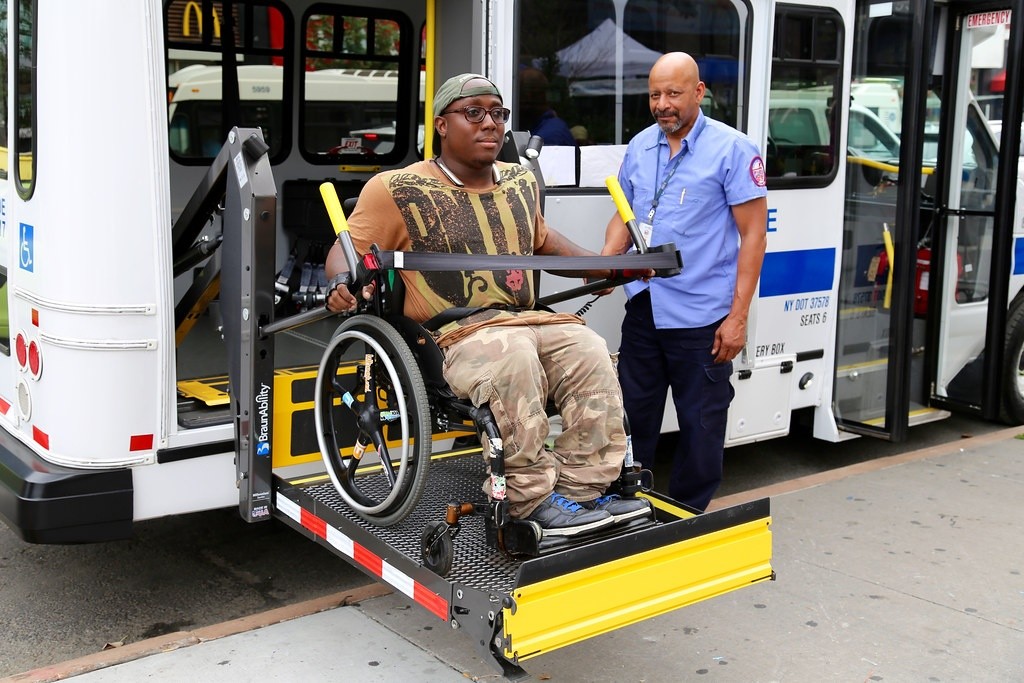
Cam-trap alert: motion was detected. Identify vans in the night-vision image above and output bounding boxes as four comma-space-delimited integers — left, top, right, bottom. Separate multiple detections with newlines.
782, 83, 900, 146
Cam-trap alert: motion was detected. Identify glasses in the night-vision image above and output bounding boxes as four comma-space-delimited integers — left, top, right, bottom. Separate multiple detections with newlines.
440, 105, 510, 125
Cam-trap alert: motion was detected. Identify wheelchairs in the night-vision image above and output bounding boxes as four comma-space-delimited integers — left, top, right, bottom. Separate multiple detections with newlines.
314, 181, 683, 576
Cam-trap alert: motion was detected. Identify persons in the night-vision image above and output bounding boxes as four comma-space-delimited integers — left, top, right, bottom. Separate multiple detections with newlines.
324, 73, 656, 536
584, 52, 767, 512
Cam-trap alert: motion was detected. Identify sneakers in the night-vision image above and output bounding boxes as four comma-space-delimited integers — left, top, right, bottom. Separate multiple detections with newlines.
576, 495, 653, 526
525, 494, 615, 536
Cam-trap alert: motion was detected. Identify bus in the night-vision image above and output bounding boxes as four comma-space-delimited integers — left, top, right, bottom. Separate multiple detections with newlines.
167, 64, 426, 158
0, 0, 1023, 545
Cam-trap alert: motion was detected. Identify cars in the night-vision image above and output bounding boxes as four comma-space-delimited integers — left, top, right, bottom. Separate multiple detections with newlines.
976, 95, 1024, 156
769, 99, 900, 163
327, 121, 426, 157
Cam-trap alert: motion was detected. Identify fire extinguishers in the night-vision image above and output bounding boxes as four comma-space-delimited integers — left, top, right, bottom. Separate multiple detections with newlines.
911, 237, 963, 319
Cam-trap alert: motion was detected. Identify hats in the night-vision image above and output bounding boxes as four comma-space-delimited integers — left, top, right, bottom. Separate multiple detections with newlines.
432, 73, 504, 120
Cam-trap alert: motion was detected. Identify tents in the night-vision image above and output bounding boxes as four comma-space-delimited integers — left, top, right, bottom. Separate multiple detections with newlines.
531, 14, 666, 87
